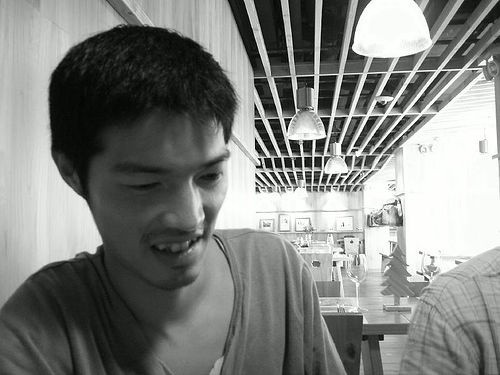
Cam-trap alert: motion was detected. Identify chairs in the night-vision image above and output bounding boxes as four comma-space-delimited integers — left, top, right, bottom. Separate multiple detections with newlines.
302, 252, 363, 375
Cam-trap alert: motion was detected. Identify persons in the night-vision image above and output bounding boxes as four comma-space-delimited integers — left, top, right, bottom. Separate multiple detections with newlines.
1, 23, 348, 375
399, 245, 500, 375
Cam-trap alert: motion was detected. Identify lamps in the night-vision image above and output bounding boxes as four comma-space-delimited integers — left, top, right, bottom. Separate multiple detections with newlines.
324, 141, 349, 175
287, 85, 326, 141
483, 61, 500, 83
351, 0, 432, 57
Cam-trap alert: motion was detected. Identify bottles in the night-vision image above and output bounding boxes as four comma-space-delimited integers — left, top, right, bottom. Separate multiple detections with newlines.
327, 234, 333, 247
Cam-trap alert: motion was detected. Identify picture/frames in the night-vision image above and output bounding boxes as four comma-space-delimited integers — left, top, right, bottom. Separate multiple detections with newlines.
278, 213, 290, 232
294, 217, 311, 231
259, 219, 274, 232
335, 216, 354, 232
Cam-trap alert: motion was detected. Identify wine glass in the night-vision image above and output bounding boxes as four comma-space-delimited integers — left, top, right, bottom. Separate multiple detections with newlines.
345, 254, 368, 313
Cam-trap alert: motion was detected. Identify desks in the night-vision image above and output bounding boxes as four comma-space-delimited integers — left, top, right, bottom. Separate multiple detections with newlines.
313, 252, 349, 297
319, 297, 417, 375
